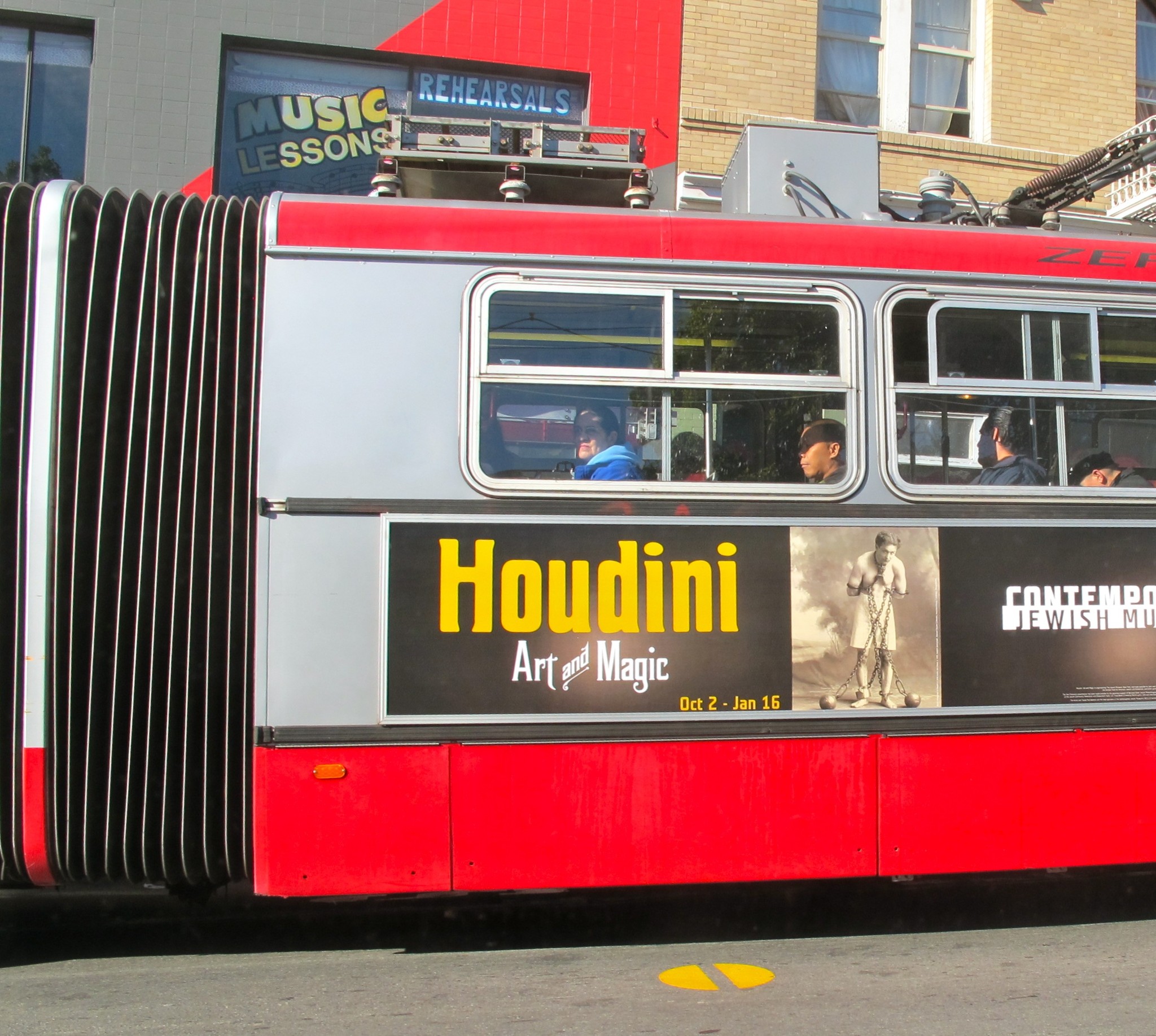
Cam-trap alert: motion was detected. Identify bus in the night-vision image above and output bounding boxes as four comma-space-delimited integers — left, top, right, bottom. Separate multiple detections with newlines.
3, 171, 1156, 904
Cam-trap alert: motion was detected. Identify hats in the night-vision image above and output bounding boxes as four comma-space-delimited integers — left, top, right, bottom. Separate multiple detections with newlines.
1069, 446, 1114, 487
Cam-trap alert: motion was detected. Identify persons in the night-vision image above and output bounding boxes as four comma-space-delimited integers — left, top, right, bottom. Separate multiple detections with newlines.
479, 415, 508, 478
570, 404, 646, 481
967, 406, 1048, 486
796, 418, 847, 485
671, 432, 706, 482
1067, 447, 1154, 488
846, 531, 909, 709
